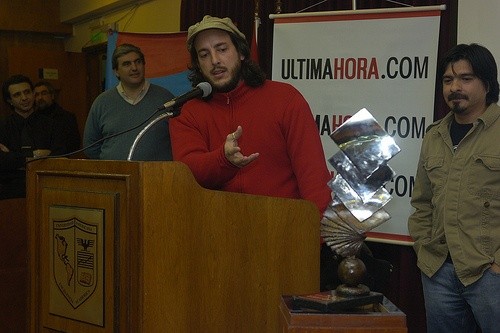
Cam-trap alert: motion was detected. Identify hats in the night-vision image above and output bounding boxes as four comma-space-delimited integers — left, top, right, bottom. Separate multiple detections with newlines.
187, 15, 246, 56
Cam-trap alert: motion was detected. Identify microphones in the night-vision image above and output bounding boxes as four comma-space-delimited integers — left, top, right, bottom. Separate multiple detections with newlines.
158, 82, 212, 111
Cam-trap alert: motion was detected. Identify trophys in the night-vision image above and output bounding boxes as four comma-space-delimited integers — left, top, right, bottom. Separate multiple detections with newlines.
295, 107, 401, 311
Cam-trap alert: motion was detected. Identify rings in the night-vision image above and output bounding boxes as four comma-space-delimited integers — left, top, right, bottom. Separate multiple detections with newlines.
232, 132, 237, 140
408, 41, 500, 333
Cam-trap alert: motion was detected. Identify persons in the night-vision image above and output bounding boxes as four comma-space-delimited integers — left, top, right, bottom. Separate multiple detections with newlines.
0, 77, 72, 198
29, 80, 82, 157
168, 15, 334, 249
83, 42, 176, 161
